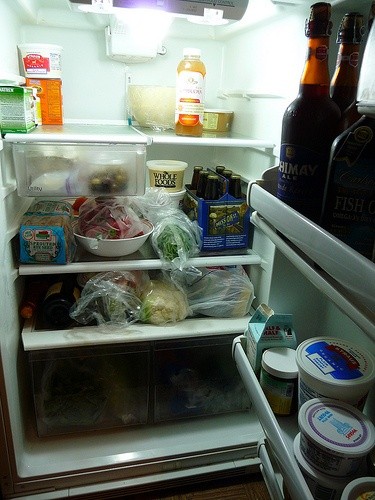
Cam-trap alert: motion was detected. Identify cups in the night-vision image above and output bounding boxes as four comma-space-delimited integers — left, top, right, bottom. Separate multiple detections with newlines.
293, 335, 375, 422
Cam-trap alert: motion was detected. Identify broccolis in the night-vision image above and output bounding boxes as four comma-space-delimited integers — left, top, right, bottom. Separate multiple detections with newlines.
137, 280, 189, 324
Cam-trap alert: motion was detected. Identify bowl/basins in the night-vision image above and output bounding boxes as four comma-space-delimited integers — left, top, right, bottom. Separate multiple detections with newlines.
74, 215, 154, 257
128, 85, 175, 129
291, 398, 375, 500
146, 158, 188, 212
18, 42, 65, 80
203, 108, 233, 133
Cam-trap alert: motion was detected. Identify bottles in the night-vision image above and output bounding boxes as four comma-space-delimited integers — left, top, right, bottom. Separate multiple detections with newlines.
19, 276, 80, 331
175, 46, 206, 138
277, 2, 346, 218
322, 112, 375, 260
327, 9, 366, 134
186, 164, 244, 250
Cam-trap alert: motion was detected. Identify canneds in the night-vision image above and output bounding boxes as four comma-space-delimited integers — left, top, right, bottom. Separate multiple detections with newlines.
173, 48, 234, 137
